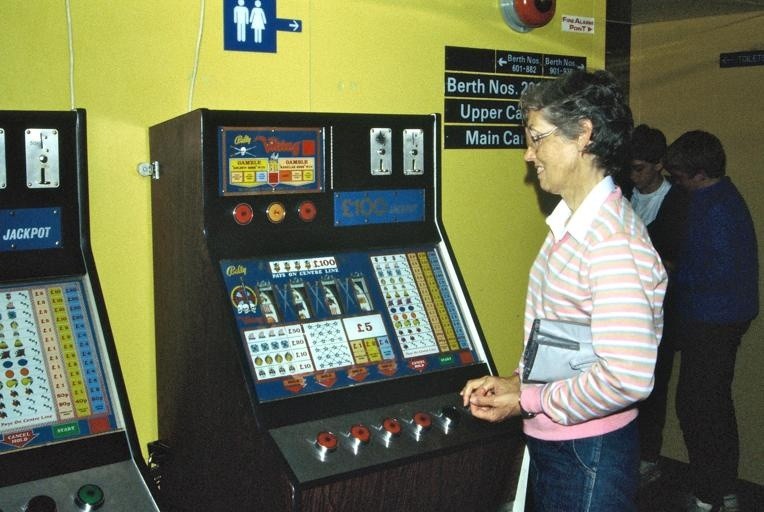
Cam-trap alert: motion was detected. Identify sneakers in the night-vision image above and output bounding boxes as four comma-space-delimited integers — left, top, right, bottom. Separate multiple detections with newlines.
685, 492, 740, 512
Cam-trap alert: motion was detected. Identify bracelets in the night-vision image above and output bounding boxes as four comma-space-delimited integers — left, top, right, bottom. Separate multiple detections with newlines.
517, 392, 537, 420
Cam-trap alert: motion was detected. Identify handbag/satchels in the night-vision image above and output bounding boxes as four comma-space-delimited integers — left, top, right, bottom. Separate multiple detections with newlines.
520, 316, 602, 385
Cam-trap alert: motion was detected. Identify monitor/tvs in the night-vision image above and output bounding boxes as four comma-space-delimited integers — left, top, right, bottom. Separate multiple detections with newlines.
0, 275, 120, 458
219, 243, 483, 404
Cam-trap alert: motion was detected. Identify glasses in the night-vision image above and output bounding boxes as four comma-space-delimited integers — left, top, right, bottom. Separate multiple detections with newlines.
523, 121, 560, 147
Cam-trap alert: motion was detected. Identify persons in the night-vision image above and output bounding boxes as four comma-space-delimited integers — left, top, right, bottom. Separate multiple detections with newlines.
456, 67, 674, 511
616, 122, 688, 492
660, 128, 760, 510
